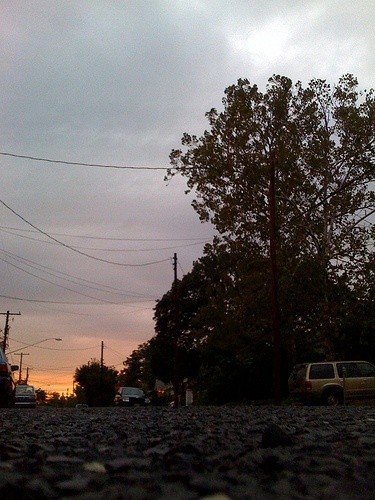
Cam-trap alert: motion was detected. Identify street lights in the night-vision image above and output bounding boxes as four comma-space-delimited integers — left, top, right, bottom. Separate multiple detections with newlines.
6, 337, 62, 355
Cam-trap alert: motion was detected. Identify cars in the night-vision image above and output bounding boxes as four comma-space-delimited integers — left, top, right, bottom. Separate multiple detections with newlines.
114, 387, 151, 407
13, 385, 37, 409
75, 404, 88, 408
288, 360, 375, 406
